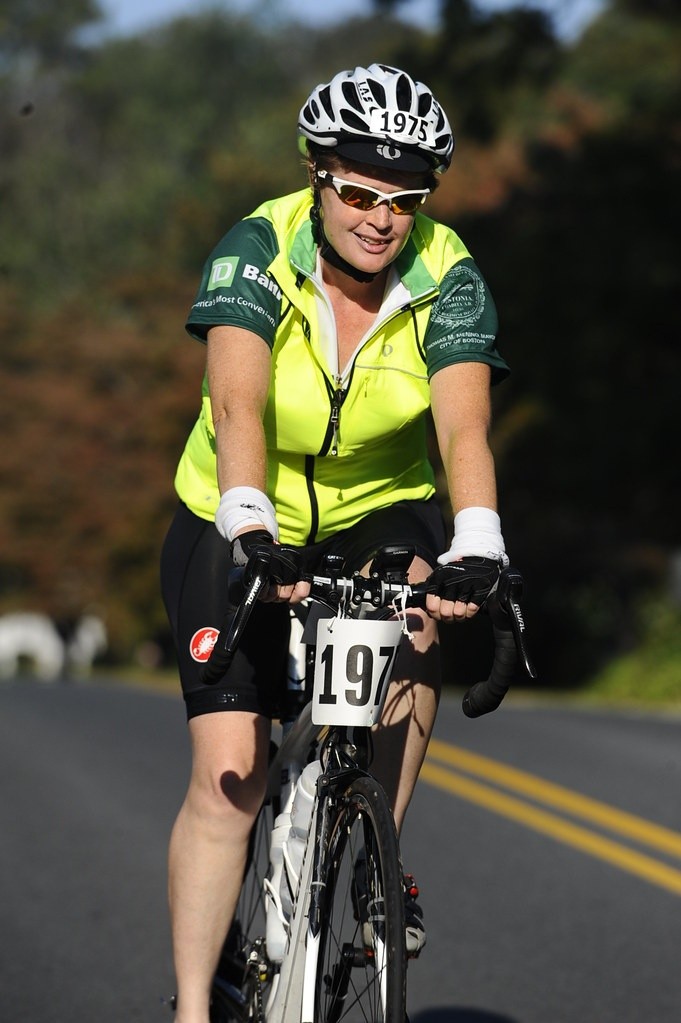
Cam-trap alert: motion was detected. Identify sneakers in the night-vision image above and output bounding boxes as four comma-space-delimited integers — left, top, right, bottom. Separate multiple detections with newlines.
351, 847, 425, 959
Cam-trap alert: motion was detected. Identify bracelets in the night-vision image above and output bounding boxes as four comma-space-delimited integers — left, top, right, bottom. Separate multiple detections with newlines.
454, 507, 501, 536
219, 486, 276, 536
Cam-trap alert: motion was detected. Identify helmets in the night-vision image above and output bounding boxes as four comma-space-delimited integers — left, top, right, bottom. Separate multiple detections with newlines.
296, 62, 453, 175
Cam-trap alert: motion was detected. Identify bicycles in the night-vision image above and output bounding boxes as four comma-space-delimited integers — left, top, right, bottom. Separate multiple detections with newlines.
171, 545, 534, 1023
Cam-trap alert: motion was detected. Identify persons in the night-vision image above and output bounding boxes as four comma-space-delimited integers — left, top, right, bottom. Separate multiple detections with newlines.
161, 62, 509, 1023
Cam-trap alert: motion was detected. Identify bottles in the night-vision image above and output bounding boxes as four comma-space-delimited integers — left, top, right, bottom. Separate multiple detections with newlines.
265, 791, 288, 965
278, 760, 325, 914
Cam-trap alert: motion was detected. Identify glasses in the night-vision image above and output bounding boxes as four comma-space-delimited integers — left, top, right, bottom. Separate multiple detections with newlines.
312, 167, 440, 215
